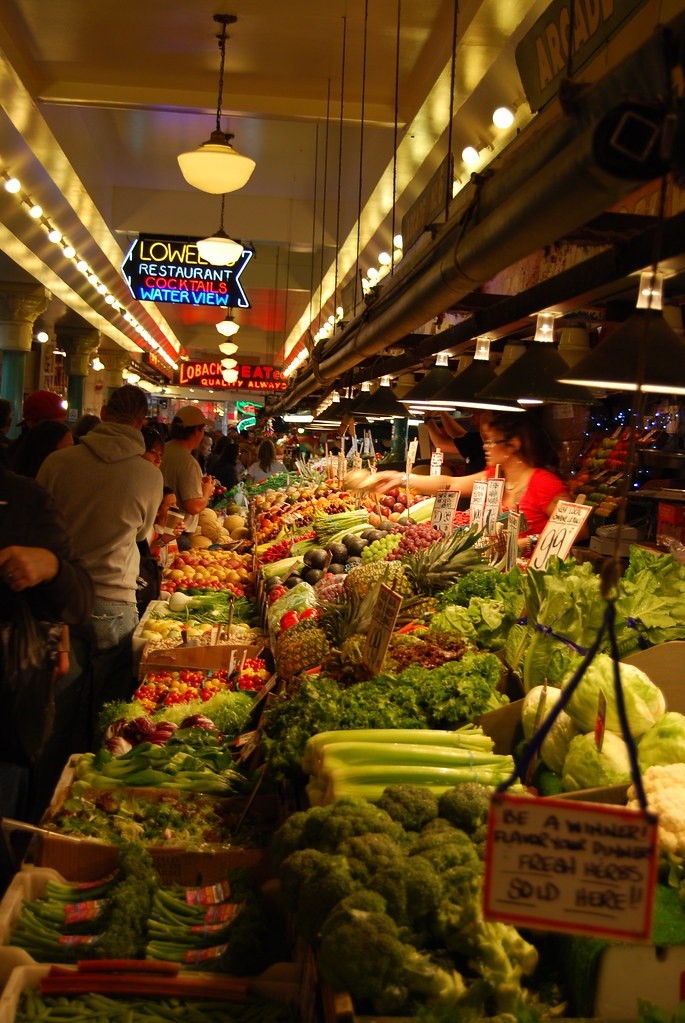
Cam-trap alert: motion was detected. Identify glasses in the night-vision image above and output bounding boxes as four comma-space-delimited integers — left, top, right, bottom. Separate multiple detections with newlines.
483, 439, 503, 448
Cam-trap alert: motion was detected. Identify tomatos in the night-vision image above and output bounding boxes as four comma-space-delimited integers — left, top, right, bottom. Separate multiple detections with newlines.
134, 655, 269, 713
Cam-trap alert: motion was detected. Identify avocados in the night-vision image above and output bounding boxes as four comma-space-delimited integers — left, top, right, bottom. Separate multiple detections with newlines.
265, 515, 417, 596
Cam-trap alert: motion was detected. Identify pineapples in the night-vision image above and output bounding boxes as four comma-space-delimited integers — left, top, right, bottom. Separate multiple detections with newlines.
276, 566, 391, 677
342, 523, 493, 603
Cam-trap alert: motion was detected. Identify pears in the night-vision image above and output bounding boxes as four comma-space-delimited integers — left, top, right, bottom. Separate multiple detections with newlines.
170, 554, 253, 571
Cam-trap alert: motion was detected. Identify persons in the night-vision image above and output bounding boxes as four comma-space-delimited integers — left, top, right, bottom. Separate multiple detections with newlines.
0, 471, 96, 819
359, 411, 590, 573
245, 440, 287, 482
422, 406, 487, 476
0, 390, 101, 477
37, 385, 164, 706
138, 406, 256, 614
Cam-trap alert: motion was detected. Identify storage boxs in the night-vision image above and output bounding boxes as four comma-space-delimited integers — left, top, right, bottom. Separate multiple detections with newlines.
131, 599, 270, 679
0, 750, 328, 1023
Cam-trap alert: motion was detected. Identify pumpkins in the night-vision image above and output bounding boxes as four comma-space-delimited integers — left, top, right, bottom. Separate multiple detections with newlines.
188, 507, 250, 549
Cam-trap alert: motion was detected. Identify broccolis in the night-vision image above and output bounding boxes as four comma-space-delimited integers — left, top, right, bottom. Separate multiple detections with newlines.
265, 782, 550, 1016
625, 762, 685, 867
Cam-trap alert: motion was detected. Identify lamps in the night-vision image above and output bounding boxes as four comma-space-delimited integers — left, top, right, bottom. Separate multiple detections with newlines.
456, 145, 487, 166
174, 1, 257, 194
193, 118, 245, 269
216, 294, 241, 383
0, 172, 120, 310
280, 233, 685, 431
492, 101, 520, 129
33, 331, 49, 343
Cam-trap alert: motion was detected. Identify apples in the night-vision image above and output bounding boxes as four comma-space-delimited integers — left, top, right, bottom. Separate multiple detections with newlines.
298, 608, 318, 622
372, 488, 427, 515
281, 610, 299, 630
569, 437, 630, 517
164, 565, 254, 585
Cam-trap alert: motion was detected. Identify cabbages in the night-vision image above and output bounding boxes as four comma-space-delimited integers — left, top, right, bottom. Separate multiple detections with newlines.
521, 652, 685, 794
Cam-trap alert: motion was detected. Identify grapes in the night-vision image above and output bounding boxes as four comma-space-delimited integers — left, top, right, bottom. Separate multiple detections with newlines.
257, 497, 352, 544
360, 523, 444, 565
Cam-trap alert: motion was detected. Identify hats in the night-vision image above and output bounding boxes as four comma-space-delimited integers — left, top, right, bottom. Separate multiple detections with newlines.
172, 406, 214, 428
23, 391, 67, 419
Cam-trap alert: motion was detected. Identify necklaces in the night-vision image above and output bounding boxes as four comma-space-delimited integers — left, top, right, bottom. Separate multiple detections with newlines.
505, 467, 530, 495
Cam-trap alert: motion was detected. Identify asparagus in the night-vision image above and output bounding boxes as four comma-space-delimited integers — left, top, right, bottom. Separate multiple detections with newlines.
311, 509, 370, 544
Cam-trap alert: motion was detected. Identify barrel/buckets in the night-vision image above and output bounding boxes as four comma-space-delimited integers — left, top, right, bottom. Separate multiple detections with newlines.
656, 501, 685, 545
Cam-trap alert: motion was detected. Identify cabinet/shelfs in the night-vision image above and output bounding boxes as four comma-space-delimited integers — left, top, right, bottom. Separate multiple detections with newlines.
561, 405, 685, 560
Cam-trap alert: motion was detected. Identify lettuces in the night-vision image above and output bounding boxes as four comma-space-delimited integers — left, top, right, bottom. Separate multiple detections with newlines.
261, 630, 511, 766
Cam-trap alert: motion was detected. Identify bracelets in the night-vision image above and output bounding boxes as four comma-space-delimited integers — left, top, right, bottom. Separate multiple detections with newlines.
155, 537, 166, 549
401, 470, 415, 488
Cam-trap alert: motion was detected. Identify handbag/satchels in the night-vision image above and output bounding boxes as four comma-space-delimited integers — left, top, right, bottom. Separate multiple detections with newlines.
0, 593, 70, 764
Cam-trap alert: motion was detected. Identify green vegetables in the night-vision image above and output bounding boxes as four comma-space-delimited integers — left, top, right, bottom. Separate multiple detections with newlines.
148, 593, 258, 627
225, 470, 311, 496
430, 547, 685, 689
71, 726, 250, 797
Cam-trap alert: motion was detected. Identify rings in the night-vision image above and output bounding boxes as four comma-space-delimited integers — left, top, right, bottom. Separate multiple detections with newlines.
6, 574, 12, 581
